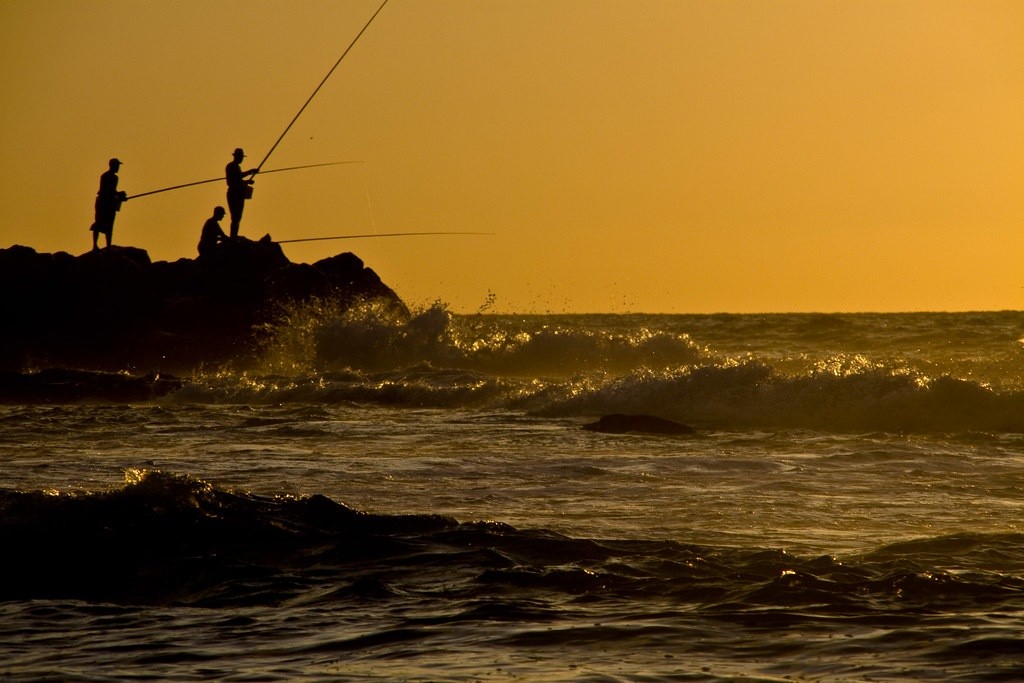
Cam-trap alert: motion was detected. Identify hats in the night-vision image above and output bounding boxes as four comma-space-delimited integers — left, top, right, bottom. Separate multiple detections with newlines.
232, 148, 246, 157
109, 158, 123, 167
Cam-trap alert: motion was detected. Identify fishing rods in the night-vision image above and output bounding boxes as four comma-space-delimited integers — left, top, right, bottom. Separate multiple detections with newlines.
125, 158, 361, 201
270, 229, 501, 242
245, 0, 388, 183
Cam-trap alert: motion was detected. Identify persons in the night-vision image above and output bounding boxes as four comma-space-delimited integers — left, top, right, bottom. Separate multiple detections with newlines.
196, 206, 227, 256
88, 159, 127, 248
225, 148, 258, 237
